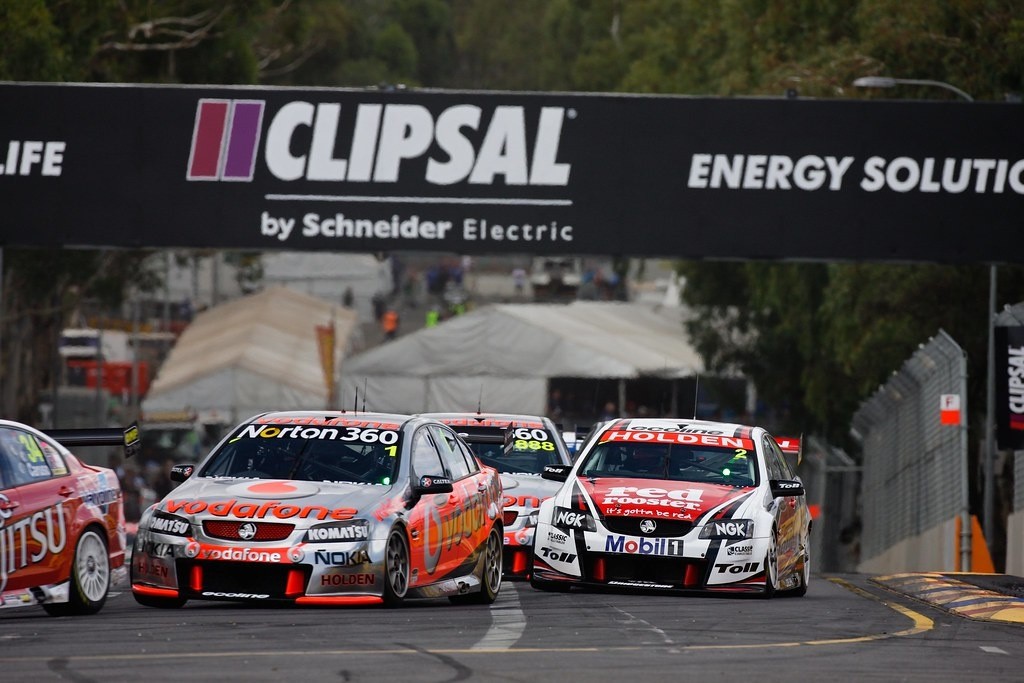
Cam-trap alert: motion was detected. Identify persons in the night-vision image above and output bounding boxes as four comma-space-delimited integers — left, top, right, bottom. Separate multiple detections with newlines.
338, 252, 694, 343
548, 387, 581, 433
598, 399, 617, 421
635, 404, 652, 418
106, 425, 203, 526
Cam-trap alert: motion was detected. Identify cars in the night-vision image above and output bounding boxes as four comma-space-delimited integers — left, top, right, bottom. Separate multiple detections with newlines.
406, 412, 574, 576
528, 417, 815, 598
0, 418, 127, 615
130, 409, 504, 609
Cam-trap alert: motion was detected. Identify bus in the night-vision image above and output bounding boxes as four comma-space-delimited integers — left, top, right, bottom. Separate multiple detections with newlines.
60, 329, 180, 408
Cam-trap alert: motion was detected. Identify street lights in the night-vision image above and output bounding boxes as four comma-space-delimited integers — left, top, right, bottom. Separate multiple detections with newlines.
852, 75, 997, 553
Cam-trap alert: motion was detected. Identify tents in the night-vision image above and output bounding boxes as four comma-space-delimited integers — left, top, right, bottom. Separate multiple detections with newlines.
257, 248, 398, 329
340, 299, 760, 434
121, 248, 244, 328
139, 287, 358, 426
